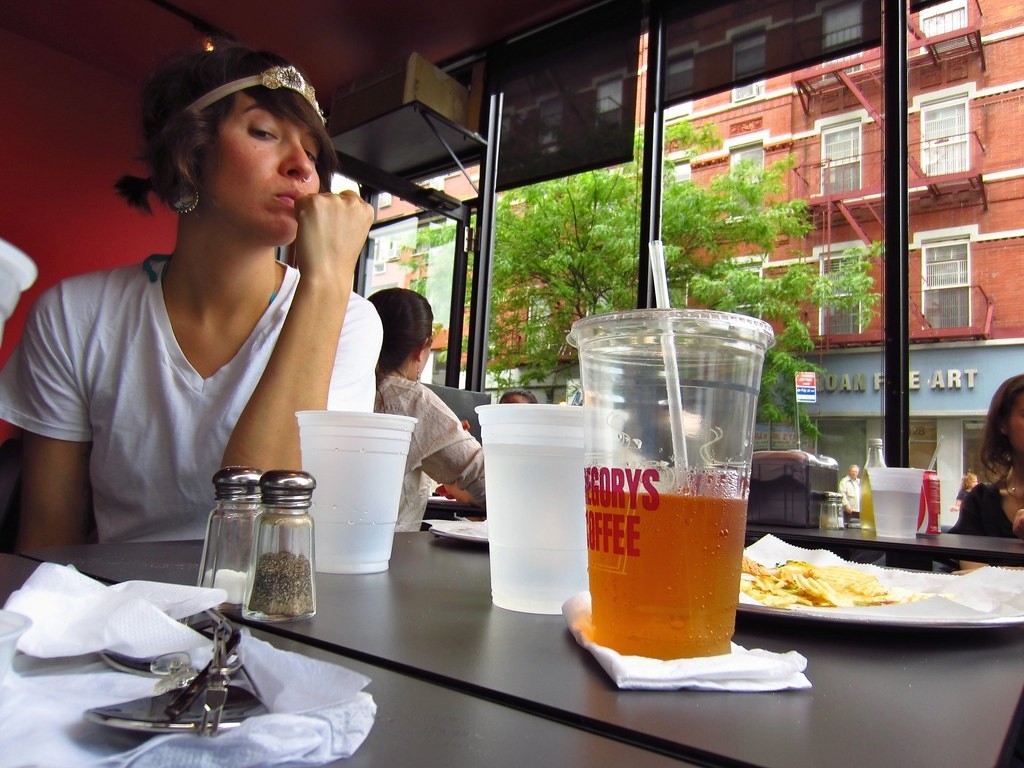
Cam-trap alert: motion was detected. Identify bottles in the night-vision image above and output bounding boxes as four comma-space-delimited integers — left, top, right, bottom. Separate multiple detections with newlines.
860, 437, 887, 530
241, 469, 319, 621
819, 492, 840, 529
834, 493, 845, 529
196, 465, 272, 614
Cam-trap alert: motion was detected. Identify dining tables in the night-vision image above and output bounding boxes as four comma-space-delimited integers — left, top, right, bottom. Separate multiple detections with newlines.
0, 532, 1023, 767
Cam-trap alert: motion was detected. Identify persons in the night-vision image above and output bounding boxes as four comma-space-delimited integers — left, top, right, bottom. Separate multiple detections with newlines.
947, 473, 978, 510
497, 389, 538, 404
0, 48, 384, 544
361, 288, 487, 532
839, 464, 860, 528
947, 374, 1024, 574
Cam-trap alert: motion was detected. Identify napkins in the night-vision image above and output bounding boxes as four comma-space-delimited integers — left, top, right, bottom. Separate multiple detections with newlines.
0, 559, 380, 768
561, 591, 812, 695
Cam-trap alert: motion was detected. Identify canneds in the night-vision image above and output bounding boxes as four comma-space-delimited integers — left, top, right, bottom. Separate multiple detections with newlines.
916, 470, 942, 539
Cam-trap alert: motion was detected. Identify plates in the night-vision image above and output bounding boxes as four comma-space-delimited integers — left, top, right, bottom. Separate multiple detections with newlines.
427, 523, 490, 546
732, 562, 1024, 629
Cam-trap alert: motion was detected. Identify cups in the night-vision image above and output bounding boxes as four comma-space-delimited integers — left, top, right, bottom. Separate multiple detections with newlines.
292, 409, 419, 575
564, 307, 777, 662
474, 403, 615, 617
864, 466, 926, 538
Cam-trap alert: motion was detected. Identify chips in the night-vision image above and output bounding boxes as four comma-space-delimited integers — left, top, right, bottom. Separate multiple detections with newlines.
742, 556, 910, 607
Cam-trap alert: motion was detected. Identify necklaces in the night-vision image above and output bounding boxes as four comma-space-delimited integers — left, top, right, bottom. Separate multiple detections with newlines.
1009, 476, 1018, 496
